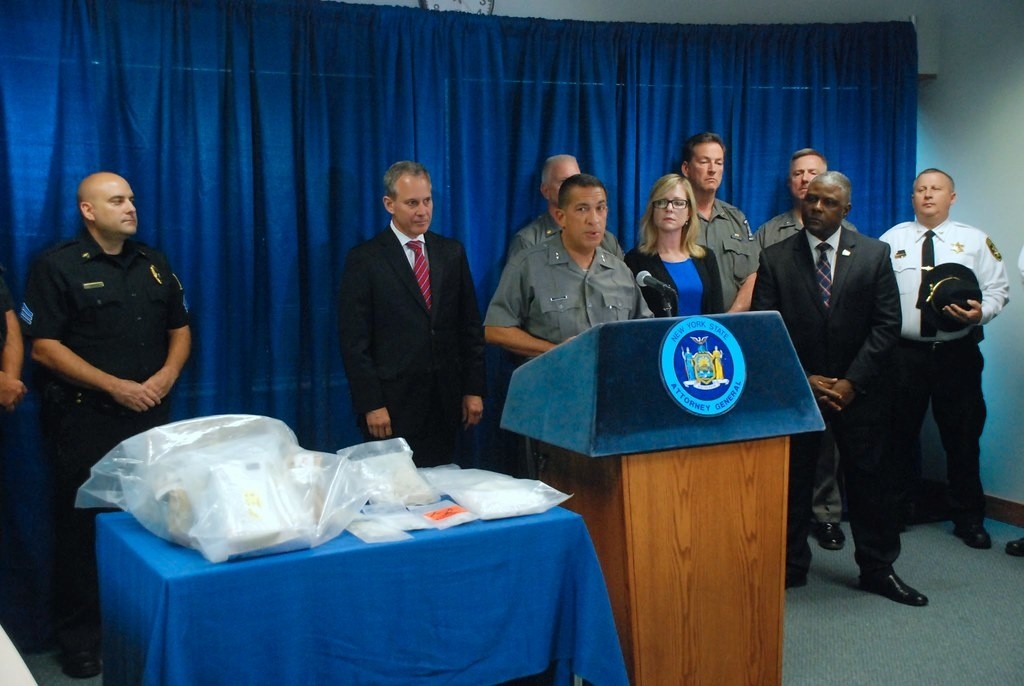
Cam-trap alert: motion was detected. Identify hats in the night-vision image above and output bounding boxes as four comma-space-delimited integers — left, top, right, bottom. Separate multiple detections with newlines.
920, 263, 982, 332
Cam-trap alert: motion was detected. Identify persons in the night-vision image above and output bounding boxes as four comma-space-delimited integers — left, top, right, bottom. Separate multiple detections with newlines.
483, 171, 654, 357
624, 172, 725, 317
681, 132, 762, 313
17, 170, 192, 678
750, 171, 928, 606
754, 149, 858, 550
338, 160, 486, 468
1004, 246, 1024, 557
0, 267, 28, 411
879, 169, 1009, 549
507, 154, 624, 263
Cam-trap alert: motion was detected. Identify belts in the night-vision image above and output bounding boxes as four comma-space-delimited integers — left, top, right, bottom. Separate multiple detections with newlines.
69, 387, 136, 420
899, 329, 976, 355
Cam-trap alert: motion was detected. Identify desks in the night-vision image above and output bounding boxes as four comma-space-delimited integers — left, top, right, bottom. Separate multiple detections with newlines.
95, 497, 627, 686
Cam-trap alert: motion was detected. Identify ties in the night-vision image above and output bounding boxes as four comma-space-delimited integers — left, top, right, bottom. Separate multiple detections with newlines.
406, 241, 432, 311
815, 242, 834, 316
920, 230, 937, 337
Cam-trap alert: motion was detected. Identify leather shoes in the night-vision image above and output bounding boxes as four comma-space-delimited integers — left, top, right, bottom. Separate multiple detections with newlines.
860, 568, 928, 605
954, 522, 991, 549
1006, 538, 1024, 556
893, 497, 921, 533
813, 521, 845, 550
785, 568, 806, 589
63, 645, 101, 677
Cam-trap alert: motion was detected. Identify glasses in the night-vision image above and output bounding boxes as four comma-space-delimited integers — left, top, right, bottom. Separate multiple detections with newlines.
652, 199, 689, 209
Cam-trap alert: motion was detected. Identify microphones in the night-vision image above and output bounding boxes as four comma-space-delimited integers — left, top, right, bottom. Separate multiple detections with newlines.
635, 270, 675, 294
